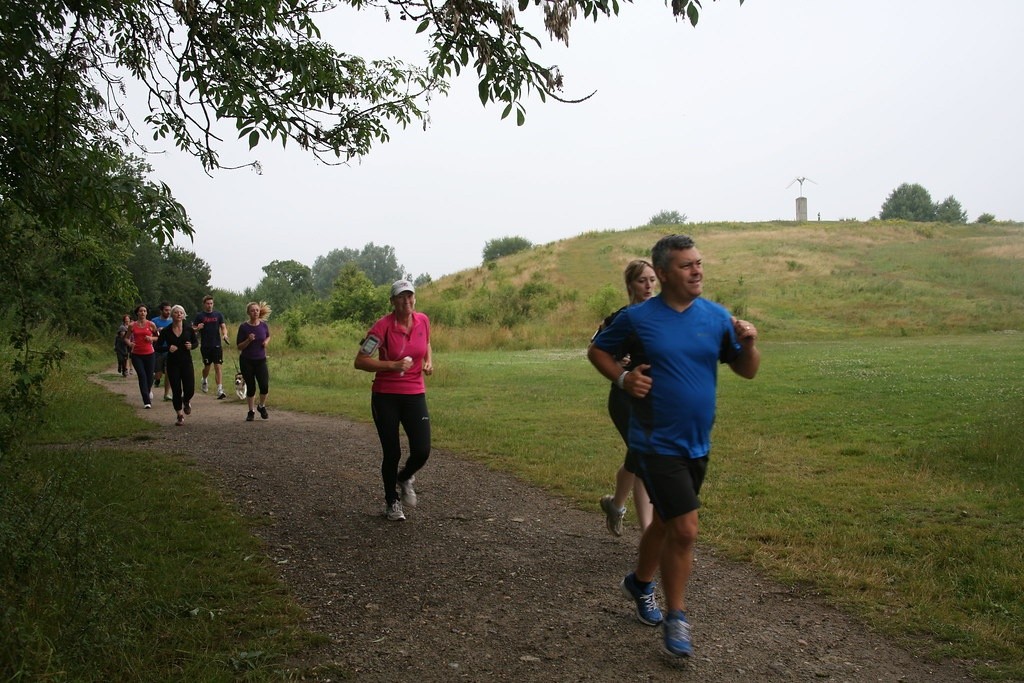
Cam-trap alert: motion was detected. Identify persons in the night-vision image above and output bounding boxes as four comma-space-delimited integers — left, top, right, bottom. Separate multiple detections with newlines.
588, 234, 759, 658
355, 279, 434, 523
190, 296, 228, 399
150, 302, 173, 402
114, 314, 134, 376
123, 305, 158, 409
156, 304, 198, 427
236, 302, 271, 422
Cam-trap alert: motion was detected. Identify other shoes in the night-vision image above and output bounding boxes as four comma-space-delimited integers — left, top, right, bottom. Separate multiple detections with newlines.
164, 394, 173, 400
117, 367, 134, 376
154, 379, 160, 385
149, 389, 153, 400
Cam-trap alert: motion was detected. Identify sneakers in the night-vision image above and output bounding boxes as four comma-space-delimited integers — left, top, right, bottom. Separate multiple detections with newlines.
144, 403, 151, 409
201, 377, 208, 392
387, 499, 406, 521
257, 404, 268, 419
175, 415, 184, 425
663, 610, 693, 658
396, 465, 416, 508
184, 400, 192, 415
600, 495, 627, 537
217, 390, 226, 399
247, 410, 255, 421
621, 573, 663, 626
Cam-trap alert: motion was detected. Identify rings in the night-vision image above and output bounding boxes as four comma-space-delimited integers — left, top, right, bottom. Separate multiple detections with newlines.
746, 325, 750, 330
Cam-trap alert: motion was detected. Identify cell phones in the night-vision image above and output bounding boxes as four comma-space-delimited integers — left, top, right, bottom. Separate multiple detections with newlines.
362, 336, 379, 355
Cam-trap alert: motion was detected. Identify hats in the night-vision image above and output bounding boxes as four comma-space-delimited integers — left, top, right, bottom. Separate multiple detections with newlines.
391, 279, 415, 297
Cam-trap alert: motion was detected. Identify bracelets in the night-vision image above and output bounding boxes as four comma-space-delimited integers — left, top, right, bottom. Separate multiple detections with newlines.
618, 368, 629, 391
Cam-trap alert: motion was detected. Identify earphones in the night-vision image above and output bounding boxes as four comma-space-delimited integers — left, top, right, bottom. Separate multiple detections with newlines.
392, 301, 394, 303
414, 299, 415, 304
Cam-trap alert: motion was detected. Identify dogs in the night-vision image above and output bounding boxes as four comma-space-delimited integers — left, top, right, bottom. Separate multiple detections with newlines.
235, 374, 248, 401
